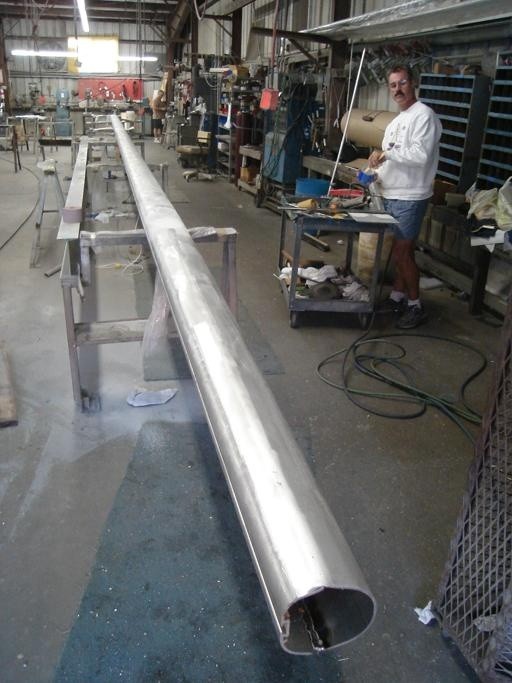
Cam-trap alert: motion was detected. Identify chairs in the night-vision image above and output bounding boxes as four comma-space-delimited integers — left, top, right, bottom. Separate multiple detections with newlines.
175, 129, 222, 183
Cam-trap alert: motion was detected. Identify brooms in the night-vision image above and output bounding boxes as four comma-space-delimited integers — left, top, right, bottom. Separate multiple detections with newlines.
300, 46, 366, 253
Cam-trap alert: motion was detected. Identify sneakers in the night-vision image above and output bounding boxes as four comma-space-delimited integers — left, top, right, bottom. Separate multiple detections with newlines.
376, 299, 428, 328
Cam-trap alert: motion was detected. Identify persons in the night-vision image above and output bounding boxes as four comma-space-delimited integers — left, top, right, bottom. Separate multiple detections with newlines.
368, 63, 442, 329
152, 90, 167, 143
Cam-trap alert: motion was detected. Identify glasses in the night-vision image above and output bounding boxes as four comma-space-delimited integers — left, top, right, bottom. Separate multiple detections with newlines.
390, 79, 407, 88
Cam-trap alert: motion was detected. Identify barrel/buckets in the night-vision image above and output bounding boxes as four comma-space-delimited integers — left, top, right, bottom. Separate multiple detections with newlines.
295, 177, 329, 196
356, 228, 395, 284
